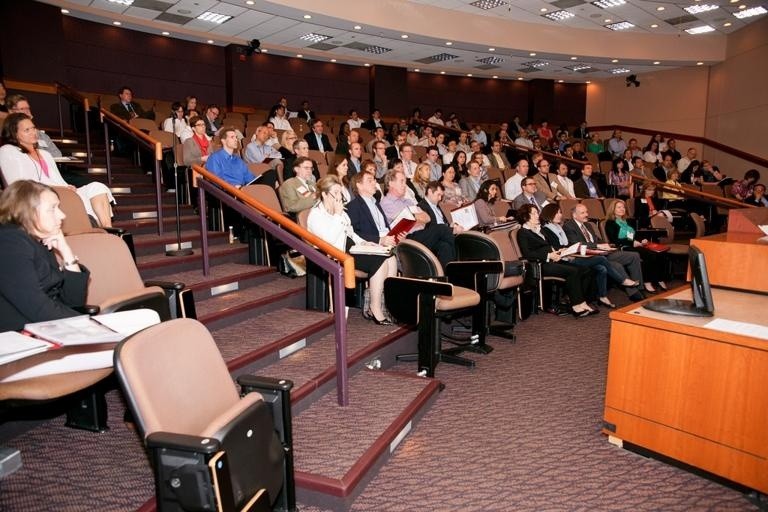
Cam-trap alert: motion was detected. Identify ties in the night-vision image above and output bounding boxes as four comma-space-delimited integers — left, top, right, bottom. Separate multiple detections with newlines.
531, 197, 536, 205
581, 225, 592, 243
126, 104, 133, 114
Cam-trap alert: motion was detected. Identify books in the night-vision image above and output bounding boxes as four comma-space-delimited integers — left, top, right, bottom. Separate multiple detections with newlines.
24, 314, 123, 347
0, 330, 54, 366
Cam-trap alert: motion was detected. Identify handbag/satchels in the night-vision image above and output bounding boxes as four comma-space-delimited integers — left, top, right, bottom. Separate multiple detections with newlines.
281, 246, 307, 278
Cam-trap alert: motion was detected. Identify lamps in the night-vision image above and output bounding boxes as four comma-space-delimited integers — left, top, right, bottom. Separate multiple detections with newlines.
246, 32, 262, 57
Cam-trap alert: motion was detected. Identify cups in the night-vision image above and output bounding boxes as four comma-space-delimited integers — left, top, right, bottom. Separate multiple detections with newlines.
580, 244, 587, 255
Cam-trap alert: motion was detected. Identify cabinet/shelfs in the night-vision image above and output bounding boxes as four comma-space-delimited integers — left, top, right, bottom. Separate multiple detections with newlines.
602, 283, 766, 494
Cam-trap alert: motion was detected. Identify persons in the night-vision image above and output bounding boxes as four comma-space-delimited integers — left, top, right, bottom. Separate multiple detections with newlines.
0, 178, 94, 331
207, 98, 726, 325
163, 95, 222, 168
0, 112, 118, 231
111, 87, 143, 122
731, 169, 767, 208
1, 79, 9, 123
3, 94, 63, 158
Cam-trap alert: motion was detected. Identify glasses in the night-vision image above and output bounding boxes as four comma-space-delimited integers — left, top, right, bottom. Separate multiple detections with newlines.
542, 164, 550, 167
432, 154, 439, 157
193, 124, 206, 127
528, 182, 536, 185
303, 166, 314, 171
328, 192, 348, 203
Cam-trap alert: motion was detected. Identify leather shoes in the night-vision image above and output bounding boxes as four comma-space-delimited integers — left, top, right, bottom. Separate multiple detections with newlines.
598, 299, 617, 309
618, 280, 640, 289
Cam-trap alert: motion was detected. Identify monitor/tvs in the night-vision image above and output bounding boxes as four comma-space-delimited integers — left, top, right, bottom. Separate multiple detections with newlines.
641, 245, 714, 317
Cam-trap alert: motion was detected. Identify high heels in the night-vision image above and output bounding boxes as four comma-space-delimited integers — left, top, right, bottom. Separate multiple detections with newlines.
368, 307, 392, 326
360, 289, 374, 320
589, 309, 600, 315
643, 284, 656, 294
572, 299, 589, 319
657, 283, 668, 291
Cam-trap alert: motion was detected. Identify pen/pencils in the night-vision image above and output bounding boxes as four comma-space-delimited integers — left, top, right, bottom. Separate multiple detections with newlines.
343, 207, 349, 211
551, 246, 557, 254
90, 317, 118, 334
507, 216, 515, 220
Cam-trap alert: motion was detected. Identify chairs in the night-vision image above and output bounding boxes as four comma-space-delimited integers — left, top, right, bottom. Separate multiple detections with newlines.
120, 64, 768, 370
0, 94, 196, 435
114, 319, 300, 512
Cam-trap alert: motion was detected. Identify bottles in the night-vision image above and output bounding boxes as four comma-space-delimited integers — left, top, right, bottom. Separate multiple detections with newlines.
228, 226, 234, 248
110, 140, 115, 153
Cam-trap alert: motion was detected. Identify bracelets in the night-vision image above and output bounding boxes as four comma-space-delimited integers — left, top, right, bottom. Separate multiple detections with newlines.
61, 255, 81, 267
